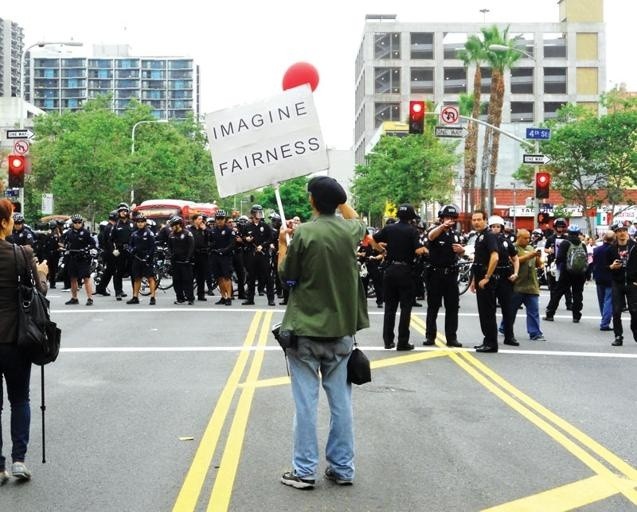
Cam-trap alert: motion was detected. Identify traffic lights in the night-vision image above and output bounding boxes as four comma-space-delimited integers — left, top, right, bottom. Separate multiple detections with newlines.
10, 201, 21, 214
232, 211, 238, 220
537, 213, 549, 224
8, 155, 25, 189
409, 100, 424, 135
536, 172, 550, 199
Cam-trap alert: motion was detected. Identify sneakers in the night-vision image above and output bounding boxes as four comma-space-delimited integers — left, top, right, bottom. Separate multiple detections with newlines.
281, 470, 315, 488
323, 465, 354, 485
0, 473, 10, 486
11, 464, 32, 480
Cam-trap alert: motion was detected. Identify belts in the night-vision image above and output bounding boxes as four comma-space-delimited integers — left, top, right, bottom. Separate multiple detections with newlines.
388, 260, 408, 265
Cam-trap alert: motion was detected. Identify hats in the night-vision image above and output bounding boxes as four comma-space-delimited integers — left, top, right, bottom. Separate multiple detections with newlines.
608, 217, 637, 232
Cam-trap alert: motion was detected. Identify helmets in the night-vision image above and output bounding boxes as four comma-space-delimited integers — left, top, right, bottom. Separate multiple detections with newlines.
204, 210, 226, 228
553, 218, 584, 235
488, 215, 504, 227
238, 204, 280, 226
108, 202, 184, 228
12, 212, 24, 222
437, 203, 460, 219
37, 213, 84, 230
395, 204, 417, 220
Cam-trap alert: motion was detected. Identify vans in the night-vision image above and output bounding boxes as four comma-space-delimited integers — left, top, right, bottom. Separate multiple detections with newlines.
38, 215, 72, 222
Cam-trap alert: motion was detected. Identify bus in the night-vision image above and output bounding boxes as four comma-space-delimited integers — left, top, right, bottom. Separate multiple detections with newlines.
133, 198, 220, 225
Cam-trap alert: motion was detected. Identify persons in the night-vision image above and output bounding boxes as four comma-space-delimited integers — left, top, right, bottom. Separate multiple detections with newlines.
1, 197, 61, 484
277, 175, 370, 489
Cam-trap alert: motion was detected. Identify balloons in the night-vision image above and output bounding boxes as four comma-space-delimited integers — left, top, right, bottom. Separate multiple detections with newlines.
283, 61, 319, 92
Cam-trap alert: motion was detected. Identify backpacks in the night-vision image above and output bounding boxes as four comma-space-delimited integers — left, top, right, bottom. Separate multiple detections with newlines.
563, 237, 589, 281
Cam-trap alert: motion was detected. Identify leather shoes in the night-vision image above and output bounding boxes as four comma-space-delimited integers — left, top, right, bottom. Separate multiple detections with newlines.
173, 299, 194, 305
504, 339, 519, 346
423, 337, 436, 345
524, 306, 632, 341
397, 343, 414, 350
611, 336, 624, 346
384, 342, 395, 348
446, 339, 462, 347
376, 293, 426, 309
473, 343, 498, 352
65, 290, 156, 306
198, 290, 287, 306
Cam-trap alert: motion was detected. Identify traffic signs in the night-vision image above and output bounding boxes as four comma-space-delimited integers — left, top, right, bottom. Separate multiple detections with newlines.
433, 125, 473, 138
523, 153, 552, 165
5, 129, 35, 139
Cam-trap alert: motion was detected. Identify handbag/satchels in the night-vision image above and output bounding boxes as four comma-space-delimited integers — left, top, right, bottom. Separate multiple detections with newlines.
13, 240, 61, 365
347, 348, 371, 386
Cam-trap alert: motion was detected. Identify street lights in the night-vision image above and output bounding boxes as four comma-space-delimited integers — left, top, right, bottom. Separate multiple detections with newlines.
19, 41, 84, 219
131, 120, 169, 213
486, 40, 542, 234
510, 183, 516, 236
365, 149, 385, 160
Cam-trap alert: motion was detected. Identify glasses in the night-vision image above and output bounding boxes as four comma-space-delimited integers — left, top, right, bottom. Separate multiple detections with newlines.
73, 221, 82, 224
136, 220, 144, 223
